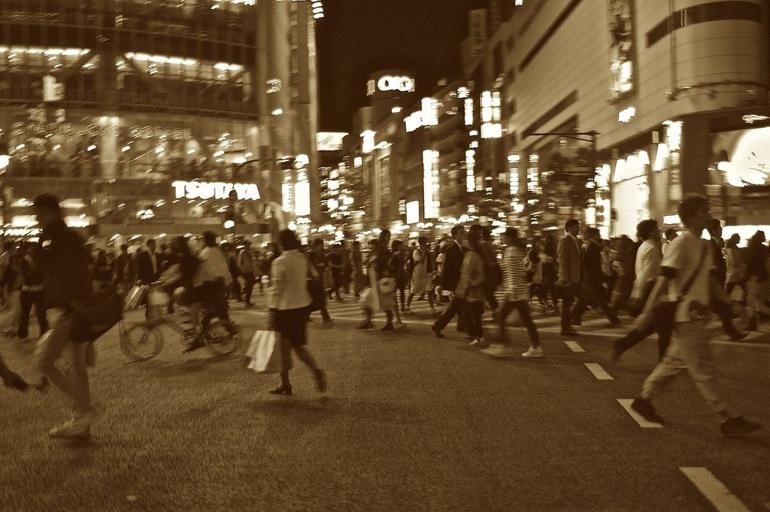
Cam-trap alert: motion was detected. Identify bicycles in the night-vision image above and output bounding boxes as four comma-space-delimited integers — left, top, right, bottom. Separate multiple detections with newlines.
116, 280, 243, 364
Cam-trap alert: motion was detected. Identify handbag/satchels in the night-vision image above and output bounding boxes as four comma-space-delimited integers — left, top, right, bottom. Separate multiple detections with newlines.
246, 331, 283, 373
653, 300, 679, 360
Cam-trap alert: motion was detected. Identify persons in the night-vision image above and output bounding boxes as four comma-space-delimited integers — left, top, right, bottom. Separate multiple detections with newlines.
631, 197, 762, 438
432, 224, 560, 358
218, 230, 452, 334
706, 219, 770, 341
556, 218, 678, 365
0, 229, 238, 392
266, 230, 328, 396
33, 194, 102, 439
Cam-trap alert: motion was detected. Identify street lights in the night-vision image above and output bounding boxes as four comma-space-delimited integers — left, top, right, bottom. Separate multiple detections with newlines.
230, 154, 310, 197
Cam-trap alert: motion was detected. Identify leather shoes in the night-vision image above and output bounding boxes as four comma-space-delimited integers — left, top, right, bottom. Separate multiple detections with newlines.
731, 331, 749, 339
269, 385, 292, 395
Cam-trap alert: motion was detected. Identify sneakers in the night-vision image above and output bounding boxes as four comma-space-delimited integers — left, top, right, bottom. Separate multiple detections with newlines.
521, 347, 543, 358
49, 420, 91, 441
469, 337, 485, 346
315, 369, 329, 394
720, 414, 761, 438
631, 396, 665, 425
395, 320, 406, 329
380, 323, 393, 331
